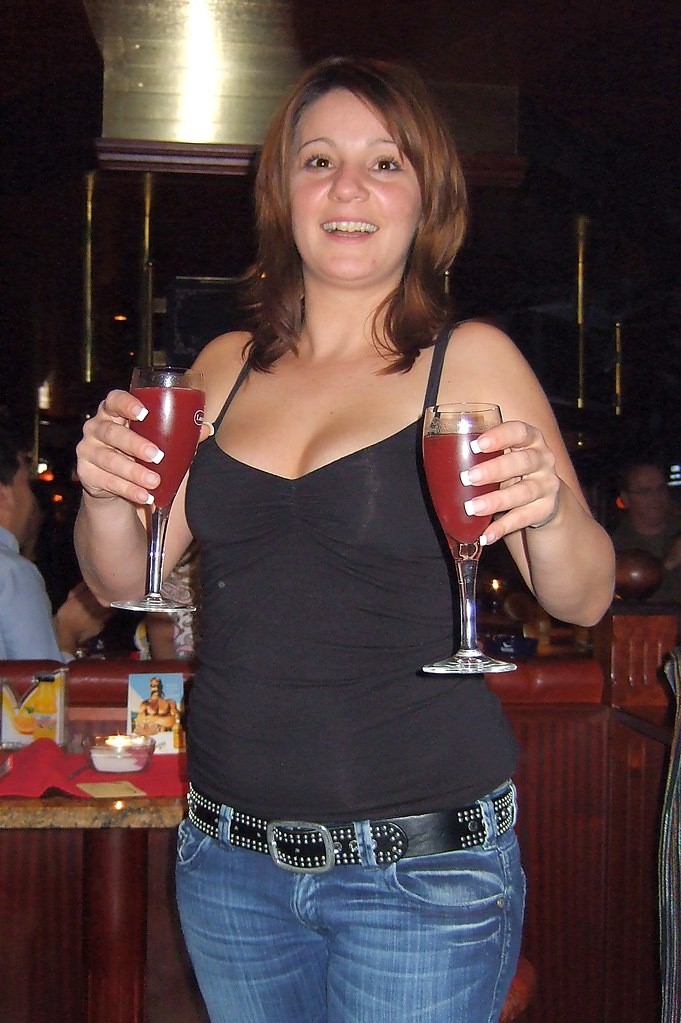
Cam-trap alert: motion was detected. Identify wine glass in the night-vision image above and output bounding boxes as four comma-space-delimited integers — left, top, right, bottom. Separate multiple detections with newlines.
109, 366, 206, 614
422, 403, 518, 673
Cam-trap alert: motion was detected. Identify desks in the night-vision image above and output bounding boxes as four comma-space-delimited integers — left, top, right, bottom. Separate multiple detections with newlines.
0, 750, 188, 1023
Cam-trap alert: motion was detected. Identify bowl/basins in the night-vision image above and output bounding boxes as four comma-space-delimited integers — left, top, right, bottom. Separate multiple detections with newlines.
88, 734, 155, 772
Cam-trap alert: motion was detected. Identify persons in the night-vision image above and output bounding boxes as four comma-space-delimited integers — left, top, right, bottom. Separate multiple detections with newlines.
74, 58, 618, 1023
608, 456, 681, 574
0, 439, 201, 666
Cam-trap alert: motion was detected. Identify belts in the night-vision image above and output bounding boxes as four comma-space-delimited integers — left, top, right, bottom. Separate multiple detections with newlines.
183, 782, 513, 875
